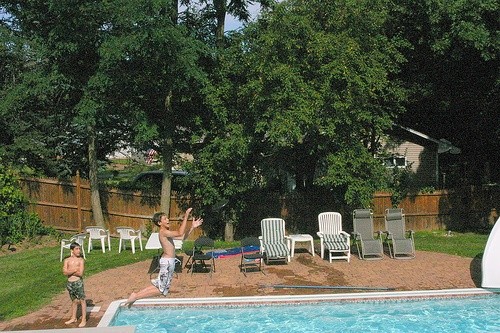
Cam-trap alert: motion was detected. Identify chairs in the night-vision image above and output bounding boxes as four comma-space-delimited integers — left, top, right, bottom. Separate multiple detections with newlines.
351, 209, 384, 260
184, 235, 215, 278
60, 233, 86, 262
385, 208, 416, 260
115, 227, 143, 254
317, 212, 351, 263
85, 226, 111, 253
259, 218, 290, 264
241, 237, 265, 277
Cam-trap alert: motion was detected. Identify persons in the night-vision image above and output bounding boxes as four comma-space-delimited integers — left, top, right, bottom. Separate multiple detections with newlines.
63, 244, 87, 328
125, 209, 204, 306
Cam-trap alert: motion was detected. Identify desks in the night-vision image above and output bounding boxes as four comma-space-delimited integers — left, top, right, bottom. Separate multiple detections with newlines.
288, 234, 314, 257
145, 233, 182, 280
